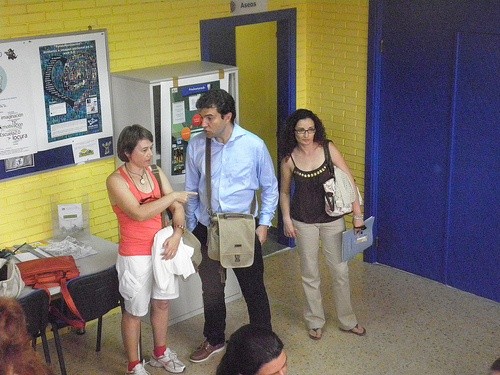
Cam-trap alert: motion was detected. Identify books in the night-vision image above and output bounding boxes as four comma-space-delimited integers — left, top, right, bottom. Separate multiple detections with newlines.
341, 216, 375, 263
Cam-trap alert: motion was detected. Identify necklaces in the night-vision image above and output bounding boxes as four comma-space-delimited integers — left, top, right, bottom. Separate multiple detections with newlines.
127, 167, 146, 185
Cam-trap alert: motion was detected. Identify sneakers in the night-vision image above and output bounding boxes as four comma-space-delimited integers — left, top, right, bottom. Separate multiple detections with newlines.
189, 340, 225, 362
150, 347, 186, 374
125, 359, 151, 375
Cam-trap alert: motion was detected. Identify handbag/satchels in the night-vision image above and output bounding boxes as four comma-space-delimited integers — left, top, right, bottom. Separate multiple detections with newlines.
17, 255, 81, 288
0, 258, 25, 299
206, 211, 256, 269
163, 219, 203, 282
322, 139, 363, 217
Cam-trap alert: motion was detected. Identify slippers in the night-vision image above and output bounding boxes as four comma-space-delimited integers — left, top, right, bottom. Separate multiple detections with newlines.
308, 328, 322, 340
340, 324, 366, 335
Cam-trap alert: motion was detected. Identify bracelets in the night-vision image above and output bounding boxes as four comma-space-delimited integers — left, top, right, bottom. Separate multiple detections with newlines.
353, 215, 364, 220
173, 225, 185, 234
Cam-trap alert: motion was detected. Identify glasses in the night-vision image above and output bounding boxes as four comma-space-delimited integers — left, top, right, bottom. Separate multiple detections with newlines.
294, 128, 317, 134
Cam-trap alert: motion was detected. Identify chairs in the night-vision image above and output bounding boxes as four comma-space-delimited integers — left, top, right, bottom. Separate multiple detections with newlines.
16, 264, 123, 375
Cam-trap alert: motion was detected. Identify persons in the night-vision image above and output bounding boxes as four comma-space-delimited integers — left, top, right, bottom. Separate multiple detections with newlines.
278, 109, 366, 340
216, 324, 288, 375
106, 124, 198, 375
185, 89, 279, 363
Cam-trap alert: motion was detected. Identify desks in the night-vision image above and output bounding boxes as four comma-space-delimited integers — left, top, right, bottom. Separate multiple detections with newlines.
0, 228, 142, 361
